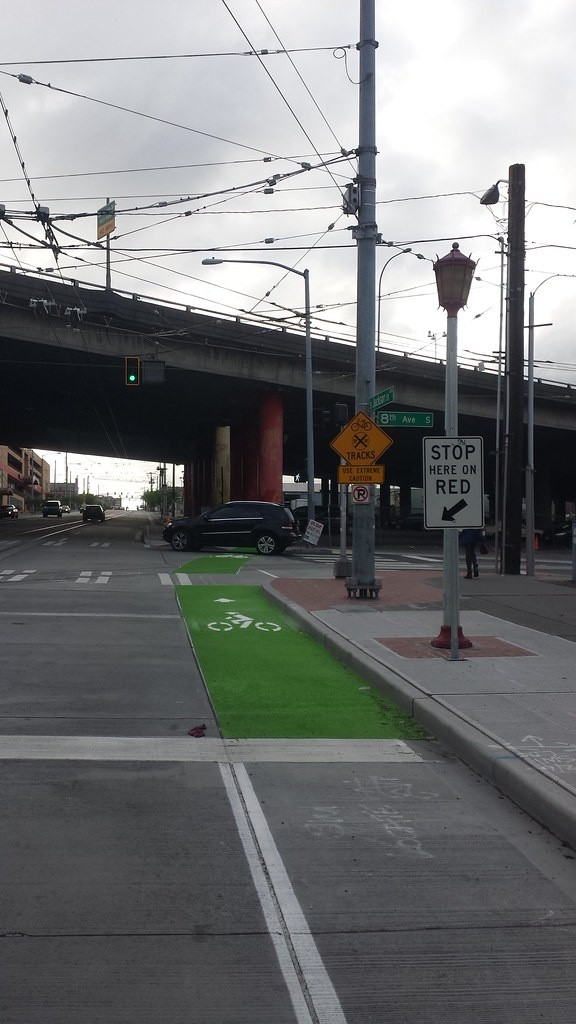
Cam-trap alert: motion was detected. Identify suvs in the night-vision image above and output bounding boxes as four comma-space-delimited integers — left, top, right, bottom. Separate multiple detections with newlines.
43, 500, 63, 518
82, 504, 106, 524
162, 500, 302, 557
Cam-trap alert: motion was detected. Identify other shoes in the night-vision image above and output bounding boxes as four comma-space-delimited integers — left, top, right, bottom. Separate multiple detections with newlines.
474, 567, 480, 577
464, 574, 473, 579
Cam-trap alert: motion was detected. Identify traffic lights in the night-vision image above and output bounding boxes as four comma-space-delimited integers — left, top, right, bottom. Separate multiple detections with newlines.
125, 356, 140, 385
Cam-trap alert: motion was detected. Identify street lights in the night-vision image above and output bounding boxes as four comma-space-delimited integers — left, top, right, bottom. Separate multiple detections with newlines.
432, 242, 473, 652
203, 256, 316, 549
527, 273, 576, 574
480, 175, 514, 576
40, 452, 61, 501
66, 463, 82, 481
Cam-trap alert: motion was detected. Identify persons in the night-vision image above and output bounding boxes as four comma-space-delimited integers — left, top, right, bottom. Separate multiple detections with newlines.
462, 529, 480, 579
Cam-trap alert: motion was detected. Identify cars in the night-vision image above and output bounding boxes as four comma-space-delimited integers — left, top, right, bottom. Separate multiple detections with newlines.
540, 518, 573, 548
0, 505, 19, 519
80, 504, 88, 513
480, 516, 544, 542
294, 506, 352, 534
61, 505, 71, 513
